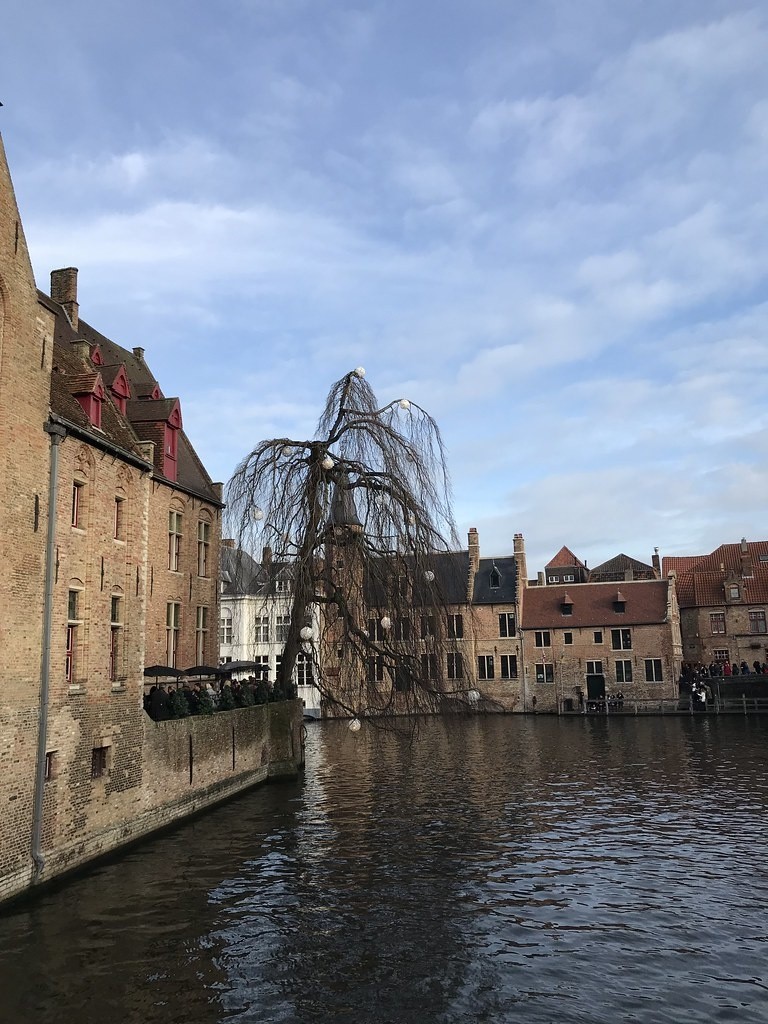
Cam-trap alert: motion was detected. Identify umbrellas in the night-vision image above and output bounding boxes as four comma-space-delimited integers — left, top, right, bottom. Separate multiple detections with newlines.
143, 659, 261, 686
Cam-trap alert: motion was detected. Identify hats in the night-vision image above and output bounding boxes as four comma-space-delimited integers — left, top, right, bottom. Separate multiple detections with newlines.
183, 681, 190, 687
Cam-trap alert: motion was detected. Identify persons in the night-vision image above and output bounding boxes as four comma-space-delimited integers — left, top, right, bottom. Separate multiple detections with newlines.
600, 690, 624, 712
144, 676, 285, 721
680, 659, 768, 711
